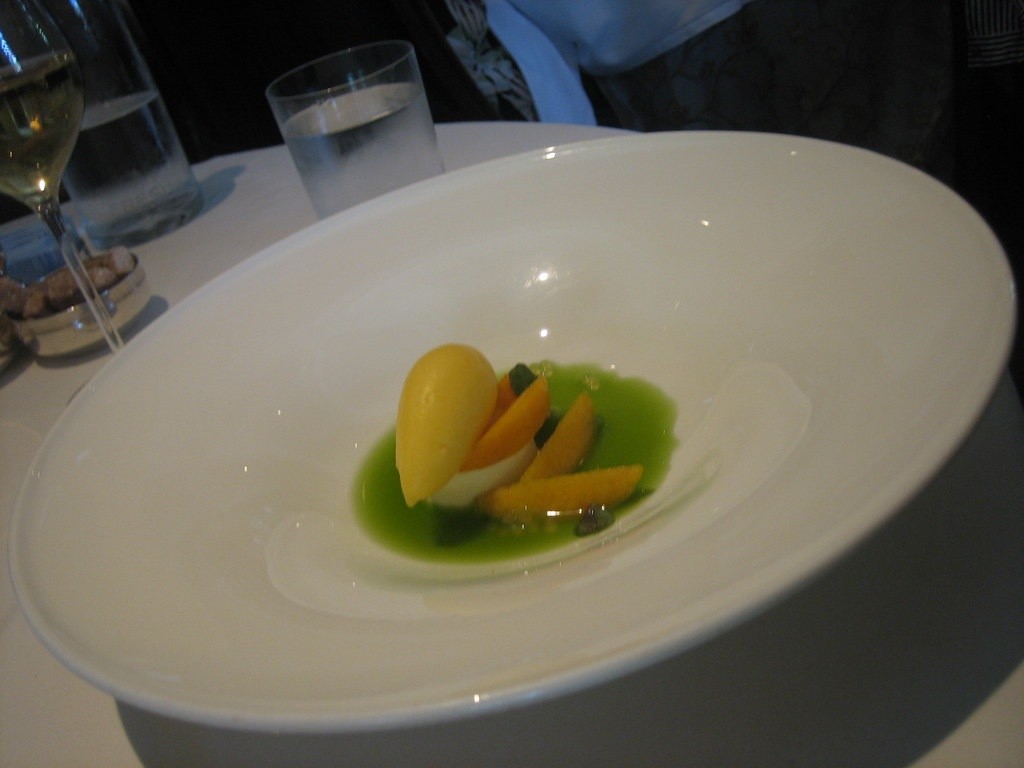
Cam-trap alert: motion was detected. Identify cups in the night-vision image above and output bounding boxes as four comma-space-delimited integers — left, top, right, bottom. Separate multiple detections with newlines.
0, 0, 205, 251
267, 40, 442, 222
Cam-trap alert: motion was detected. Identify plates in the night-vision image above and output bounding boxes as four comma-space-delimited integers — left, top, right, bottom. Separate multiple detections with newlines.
8, 129, 1016, 733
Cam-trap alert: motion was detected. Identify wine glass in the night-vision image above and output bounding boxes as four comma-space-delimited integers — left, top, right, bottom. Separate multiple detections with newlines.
1, 0, 126, 356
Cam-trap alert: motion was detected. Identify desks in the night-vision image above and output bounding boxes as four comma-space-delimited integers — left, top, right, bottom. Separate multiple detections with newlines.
0, 119, 1024, 768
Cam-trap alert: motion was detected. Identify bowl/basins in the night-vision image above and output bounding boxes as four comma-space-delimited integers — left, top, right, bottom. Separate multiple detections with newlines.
9, 247, 147, 359
